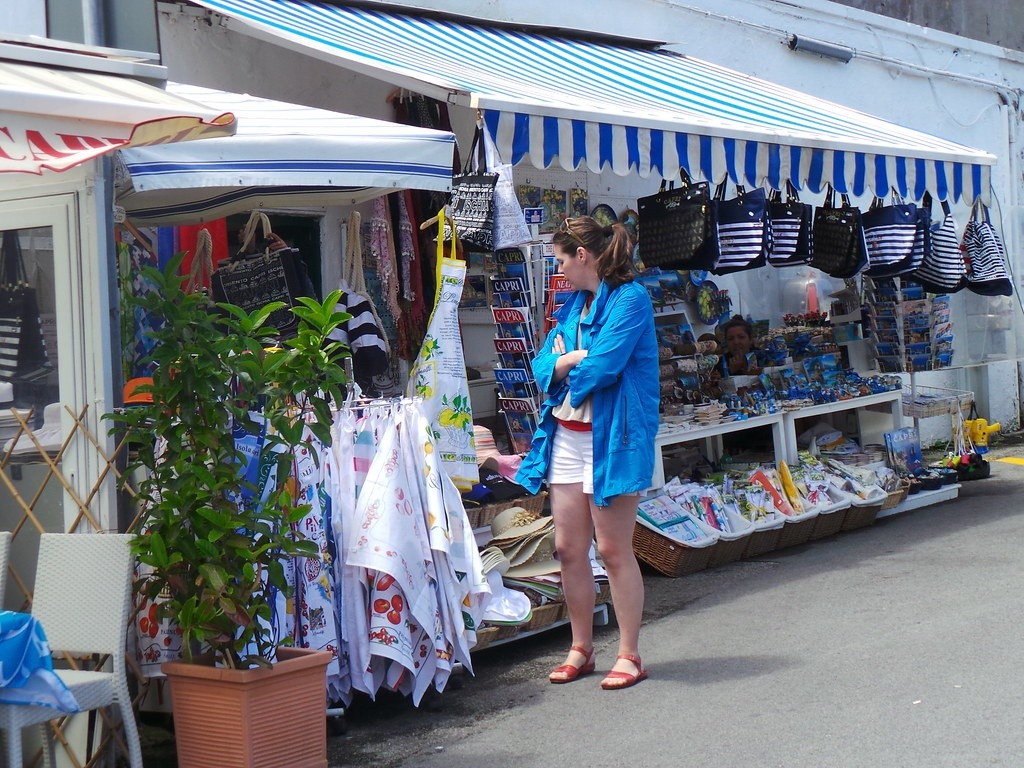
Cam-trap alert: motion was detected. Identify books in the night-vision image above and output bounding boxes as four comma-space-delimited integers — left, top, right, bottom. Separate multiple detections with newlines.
884, 429, 922, 475
864, 273, 957, 373
488, 240, 574, 457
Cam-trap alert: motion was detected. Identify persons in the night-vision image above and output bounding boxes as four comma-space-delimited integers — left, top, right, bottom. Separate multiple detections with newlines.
526, 208, 658, 690
713, 317, 769, 376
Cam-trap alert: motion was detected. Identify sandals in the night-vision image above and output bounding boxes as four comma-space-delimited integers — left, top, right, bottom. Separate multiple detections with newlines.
600, 655, 648, 689
548, 645, 597, 684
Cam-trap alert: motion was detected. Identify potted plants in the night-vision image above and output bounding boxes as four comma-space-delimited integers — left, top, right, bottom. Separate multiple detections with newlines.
101, 250, 353, 768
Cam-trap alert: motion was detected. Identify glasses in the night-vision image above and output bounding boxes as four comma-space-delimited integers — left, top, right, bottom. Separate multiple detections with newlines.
560, 217, 592, 253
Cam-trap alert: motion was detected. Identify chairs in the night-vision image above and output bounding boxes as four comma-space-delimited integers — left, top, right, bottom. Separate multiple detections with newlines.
1, 532, 144, 768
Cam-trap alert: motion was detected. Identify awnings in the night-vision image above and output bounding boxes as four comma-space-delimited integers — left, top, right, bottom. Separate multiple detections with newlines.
193, 0, 1001, 206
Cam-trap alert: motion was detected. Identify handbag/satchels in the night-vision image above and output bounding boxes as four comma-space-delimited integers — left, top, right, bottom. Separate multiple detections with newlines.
0, 231, 57, 387
312, 209, 390, 384
213, 206, 325, 349
432, 114, 535, 258
959, 197, 1016, 301
635, 168, 968, 295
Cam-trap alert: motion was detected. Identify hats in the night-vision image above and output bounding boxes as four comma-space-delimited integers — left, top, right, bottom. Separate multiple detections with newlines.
477, 507, 563, 626
472, 424, 502, 473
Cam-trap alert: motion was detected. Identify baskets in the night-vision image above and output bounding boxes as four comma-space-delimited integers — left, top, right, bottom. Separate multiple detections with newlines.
831, 482, 888, 532
879, 488, 905, 510
472, 626, 498, 651
900, 478, 911, 501
631, 514, 721, 578
808, 483, 851, 541
461, 497, 482, 529
520, 602, 564, 631
479, 501, 517, 529
594, 584, 612, 605
776, 496, 820, 551
513, 489, 549, 516
493, 624, 526, 640
865, 385, 976, 418
680, 502, 756, 570
745, 508, 787, 560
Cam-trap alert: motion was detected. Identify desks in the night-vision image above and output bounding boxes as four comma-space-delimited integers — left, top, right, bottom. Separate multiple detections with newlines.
651, 412, 788, 488
712, 389, 904, 466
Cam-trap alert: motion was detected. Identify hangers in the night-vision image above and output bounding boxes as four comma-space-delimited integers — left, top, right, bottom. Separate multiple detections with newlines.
420, 195, 463, 253
